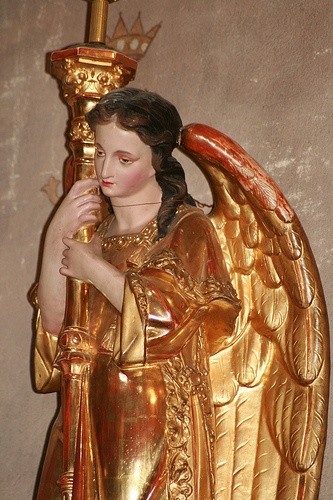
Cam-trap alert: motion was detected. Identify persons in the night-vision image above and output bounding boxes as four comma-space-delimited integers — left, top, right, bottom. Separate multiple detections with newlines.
29, 86, 241, 500
